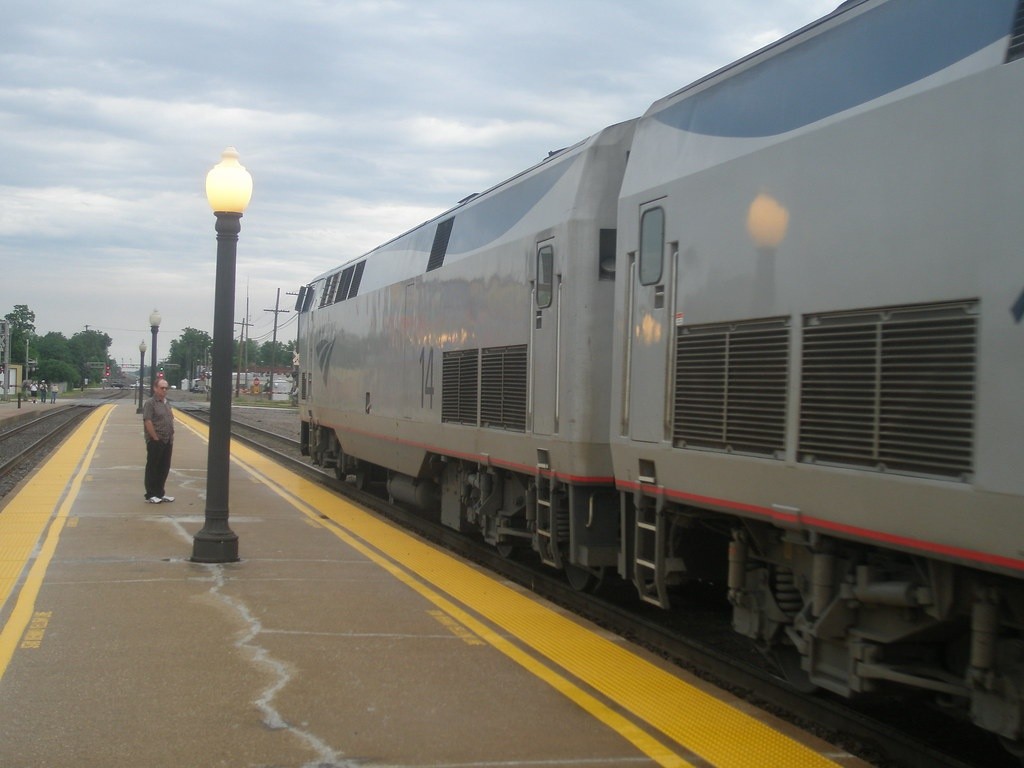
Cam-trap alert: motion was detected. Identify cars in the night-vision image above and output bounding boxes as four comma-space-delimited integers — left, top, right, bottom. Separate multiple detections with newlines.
109, 381, 210, 395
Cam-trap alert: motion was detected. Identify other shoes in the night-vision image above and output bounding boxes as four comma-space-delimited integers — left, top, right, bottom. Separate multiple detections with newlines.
35, 402, 37, 404
30, 402, 33, 404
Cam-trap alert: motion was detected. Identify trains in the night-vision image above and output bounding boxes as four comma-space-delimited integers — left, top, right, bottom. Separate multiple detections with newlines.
295, 0, 1024, 768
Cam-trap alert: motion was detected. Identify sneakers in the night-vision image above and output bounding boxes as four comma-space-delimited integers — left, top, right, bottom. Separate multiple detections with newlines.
145, 497, 162, 504
161, 496, 175, 502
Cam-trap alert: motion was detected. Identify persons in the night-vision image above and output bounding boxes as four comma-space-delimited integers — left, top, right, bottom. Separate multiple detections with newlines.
30, 380, 57, 404
143, 379, 175, 503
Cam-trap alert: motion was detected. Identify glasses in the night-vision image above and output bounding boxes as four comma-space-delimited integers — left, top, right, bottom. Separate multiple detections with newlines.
157, 386, 168, 390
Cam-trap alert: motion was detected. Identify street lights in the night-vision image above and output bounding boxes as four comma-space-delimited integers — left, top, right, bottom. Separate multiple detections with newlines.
190, 145, 253, 563
136, 338, 147, 414
747, 188, 789, 321
150, 307, 161, 397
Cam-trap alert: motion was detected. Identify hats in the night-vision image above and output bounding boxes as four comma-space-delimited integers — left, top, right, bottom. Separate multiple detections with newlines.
41, 380, 46, 382
